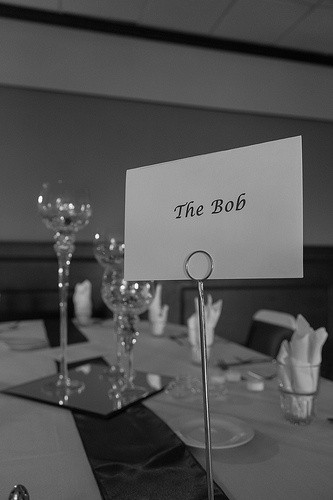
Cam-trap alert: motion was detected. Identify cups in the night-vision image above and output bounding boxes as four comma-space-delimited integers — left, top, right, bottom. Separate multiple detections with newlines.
189, 332, 213, 363
275, 359, 320, 424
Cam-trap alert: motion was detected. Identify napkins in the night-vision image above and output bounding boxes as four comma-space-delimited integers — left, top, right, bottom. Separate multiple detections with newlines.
186, 295, 224, 361
278, 313, 328, 416
74, 279, 93, 323
149, 284, 169, 334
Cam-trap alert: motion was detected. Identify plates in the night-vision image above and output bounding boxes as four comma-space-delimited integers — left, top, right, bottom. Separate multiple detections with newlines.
169, 413, 255, 450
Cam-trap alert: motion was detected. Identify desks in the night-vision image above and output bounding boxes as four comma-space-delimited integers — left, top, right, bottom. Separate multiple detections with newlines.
0, 317, 333, 500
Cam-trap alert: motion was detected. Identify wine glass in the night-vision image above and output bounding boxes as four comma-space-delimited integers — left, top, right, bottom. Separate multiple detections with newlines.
93, 234, 134, 377
101, 268, 155, 399
39, 179, 91, 395
